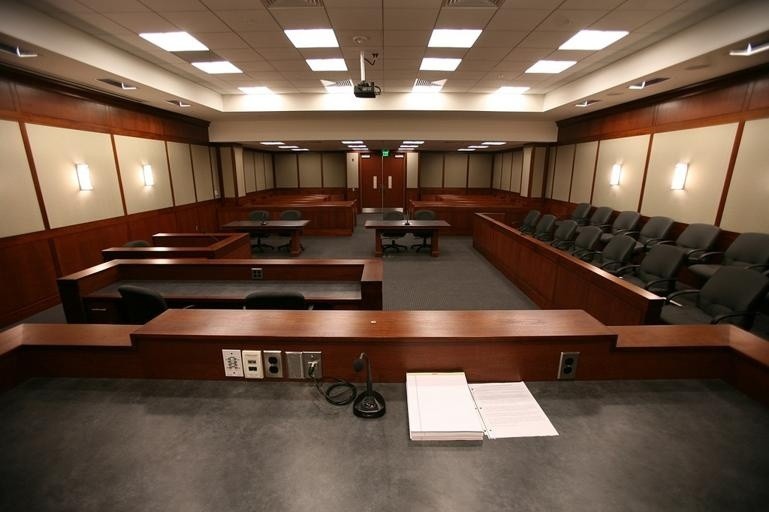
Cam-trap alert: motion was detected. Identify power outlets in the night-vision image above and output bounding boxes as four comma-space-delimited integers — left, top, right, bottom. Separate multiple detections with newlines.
222, 349, 324, 381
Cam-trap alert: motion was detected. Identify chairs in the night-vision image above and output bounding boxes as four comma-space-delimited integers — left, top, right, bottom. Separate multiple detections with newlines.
221, 210, 452, 257
241, 285, 316, 315
119, 283, 198, 319
509, 203, 769, 339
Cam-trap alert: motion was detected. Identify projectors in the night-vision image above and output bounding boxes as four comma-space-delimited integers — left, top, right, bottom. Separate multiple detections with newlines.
354, 81, 376, 98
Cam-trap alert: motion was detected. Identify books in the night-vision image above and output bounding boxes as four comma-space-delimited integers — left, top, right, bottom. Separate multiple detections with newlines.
404, 369, 486, 441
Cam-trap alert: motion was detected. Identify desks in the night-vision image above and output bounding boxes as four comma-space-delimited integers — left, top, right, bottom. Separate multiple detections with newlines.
57, 257, 382, 319
102, 233, 251, 261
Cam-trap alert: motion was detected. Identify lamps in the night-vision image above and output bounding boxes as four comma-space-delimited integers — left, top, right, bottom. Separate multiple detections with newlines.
76, 160, 156, 193
608, 161, 688, 190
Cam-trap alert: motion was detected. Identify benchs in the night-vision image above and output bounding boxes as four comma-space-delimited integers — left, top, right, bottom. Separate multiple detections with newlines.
242, 191, 356, 236
409, 193, 527, 234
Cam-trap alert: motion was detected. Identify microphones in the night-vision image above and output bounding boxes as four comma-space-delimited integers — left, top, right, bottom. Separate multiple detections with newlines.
352, 352, 385, 419
